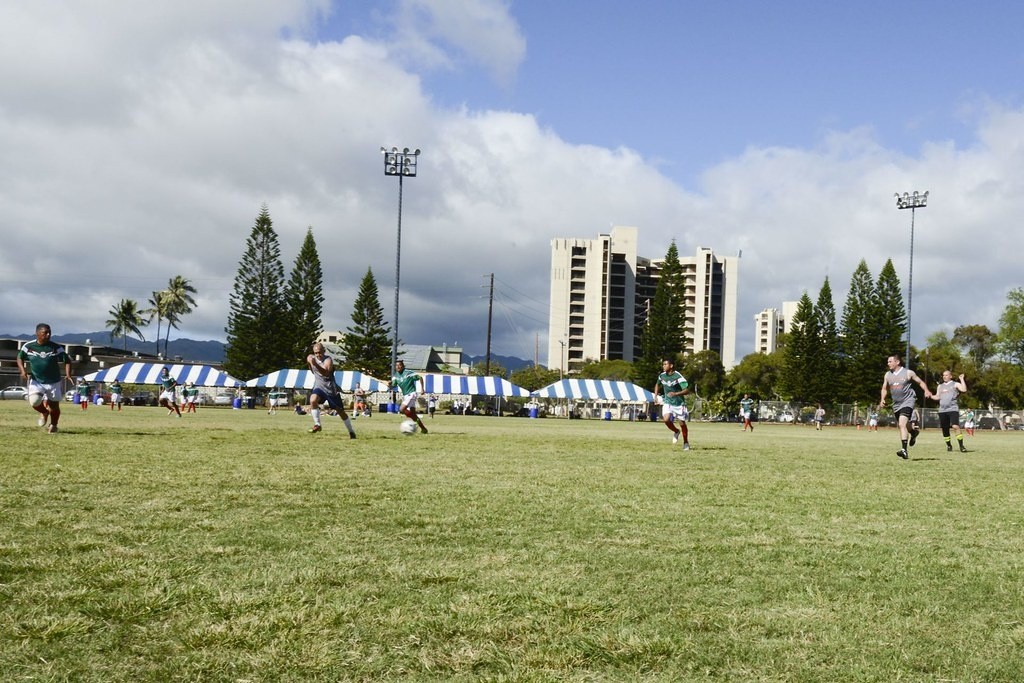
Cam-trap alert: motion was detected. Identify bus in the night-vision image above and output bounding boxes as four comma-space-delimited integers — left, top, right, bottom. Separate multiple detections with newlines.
269, 392, 289, 406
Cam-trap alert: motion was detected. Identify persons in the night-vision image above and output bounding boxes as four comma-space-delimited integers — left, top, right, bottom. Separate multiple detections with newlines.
965, 408, 975, 436
449, 398, 498, 415
739, 393, 753, 431
911, 409, 920, 429
123, 397, 158, 406
388, 362, 427, 433
814, 405, 825, 430
930, 371, 967, 452
880, 353, 931, 459
869, 411, 878, 432
640, 406, 656, 421
654, 358, 689, 450
267, 383, 372, 419
307, 343, 356, 439
159, 367, 198, 417
429, 394, 438, 418
17, 324, 75, 432
77, 377, 122, 411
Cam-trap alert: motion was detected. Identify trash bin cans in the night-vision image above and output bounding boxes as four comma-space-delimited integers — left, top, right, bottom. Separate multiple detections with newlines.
73, 393, 80, 404
93, 394, 100, 404
605, 412, 611, 419
530, 409, 538, 417
248, 399, 256, 408
387, 404, 395, 412
233, 397, 241, 408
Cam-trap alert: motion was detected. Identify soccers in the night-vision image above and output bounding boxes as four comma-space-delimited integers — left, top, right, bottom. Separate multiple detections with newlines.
400, 420, 418, 436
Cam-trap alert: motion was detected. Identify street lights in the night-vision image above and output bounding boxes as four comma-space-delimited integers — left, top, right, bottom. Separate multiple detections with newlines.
893, 191, 930, 369
380, 146, 421, 414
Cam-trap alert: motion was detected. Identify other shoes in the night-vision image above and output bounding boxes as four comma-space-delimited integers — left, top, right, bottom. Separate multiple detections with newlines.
414, 417, 419, 427
420, 429, 428, 434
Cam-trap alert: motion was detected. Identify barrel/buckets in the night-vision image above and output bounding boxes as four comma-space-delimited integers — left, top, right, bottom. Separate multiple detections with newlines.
395, 403, 400, 413
73, 394, 80, 404
530, 408, 538, 418
605, 412, 611, 420
651, 413, 656, 421
233, 398, 241, 409
248, 399, 255, 408
387, 403, 394, 413
670, 415, 674, 422
93, 394, 100, 404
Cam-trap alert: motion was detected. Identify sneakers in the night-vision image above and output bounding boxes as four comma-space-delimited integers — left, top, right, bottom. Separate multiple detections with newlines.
961, 446, 966, 452
349, 432, 355, 441
48, 425, 57, 434
38, 409, 49, 427
308, 425, 322, 433
909, 431, 919, 446
896, 448, 908, 459
947, 446, 951, 450
673, 430, 680, 444
683, 443, 689, 451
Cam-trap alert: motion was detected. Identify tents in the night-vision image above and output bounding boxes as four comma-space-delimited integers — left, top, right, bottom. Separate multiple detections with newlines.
77, 362, 247, 408
531, 378, 665, 422
391, 372, 533, 416
246, 369, 391, 408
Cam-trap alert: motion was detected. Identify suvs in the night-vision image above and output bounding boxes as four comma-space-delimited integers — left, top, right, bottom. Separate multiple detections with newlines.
64, 385, 96, 403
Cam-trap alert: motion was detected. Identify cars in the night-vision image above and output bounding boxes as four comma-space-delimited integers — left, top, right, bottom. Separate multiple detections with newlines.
0, 386, 29, 399
214, 392, 234, 406
92, 389, 131, 404
237, 390, 256, 404
796, 414, 824, 424
192, 393, 211, 404
776, 411, 794, 422
129, 390, 158, 404
